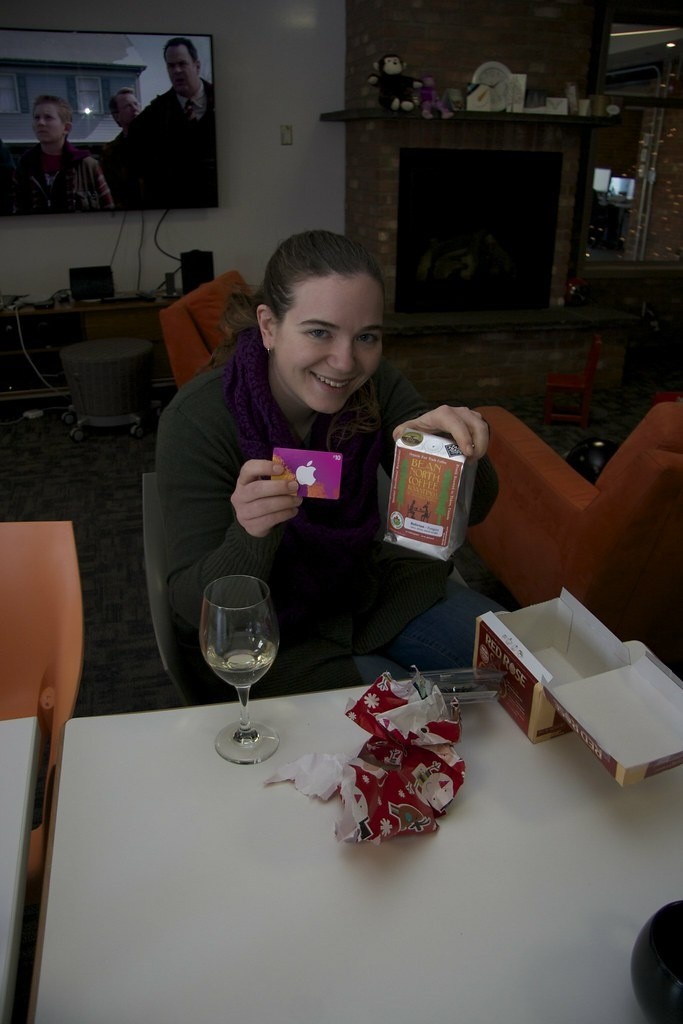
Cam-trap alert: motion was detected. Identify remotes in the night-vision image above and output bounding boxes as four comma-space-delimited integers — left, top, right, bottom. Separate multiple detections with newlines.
137, 292, 157, 302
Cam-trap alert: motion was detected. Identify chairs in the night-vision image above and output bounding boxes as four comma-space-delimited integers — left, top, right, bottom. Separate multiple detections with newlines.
544, 332, 600, 426
0, 521, 84, 874
158, 268, 255, 392
143, 471, 469, 710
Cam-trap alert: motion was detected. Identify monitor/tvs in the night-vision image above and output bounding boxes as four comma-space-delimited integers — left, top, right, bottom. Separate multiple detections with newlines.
0, 27, 219, 216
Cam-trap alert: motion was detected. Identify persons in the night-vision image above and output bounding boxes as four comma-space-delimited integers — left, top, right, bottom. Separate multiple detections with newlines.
156, 229, 521, 686
21, 37, 217, 210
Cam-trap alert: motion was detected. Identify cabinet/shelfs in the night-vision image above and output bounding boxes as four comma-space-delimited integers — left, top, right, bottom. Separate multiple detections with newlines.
0, 292, 179, 401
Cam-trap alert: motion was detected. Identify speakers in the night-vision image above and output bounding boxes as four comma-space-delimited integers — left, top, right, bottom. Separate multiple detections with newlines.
181, 251, 214, 294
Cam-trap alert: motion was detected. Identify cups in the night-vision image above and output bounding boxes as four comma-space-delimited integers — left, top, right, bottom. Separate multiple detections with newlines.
589, 95, 610, 116
579, 99, 591, 116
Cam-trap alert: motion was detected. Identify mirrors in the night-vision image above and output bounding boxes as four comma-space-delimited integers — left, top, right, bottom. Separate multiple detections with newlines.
569, 0, 683, 280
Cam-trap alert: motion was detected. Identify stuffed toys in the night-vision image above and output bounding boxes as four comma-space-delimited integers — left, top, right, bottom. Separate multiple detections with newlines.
418, 74, 454, 119
369, 56, 422, 111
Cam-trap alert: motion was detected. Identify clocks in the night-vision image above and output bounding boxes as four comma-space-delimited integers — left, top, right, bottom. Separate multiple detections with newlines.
460, 60, 511, 112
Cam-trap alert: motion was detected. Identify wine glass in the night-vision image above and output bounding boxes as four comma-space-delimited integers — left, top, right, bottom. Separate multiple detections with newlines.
199, 575, 278, 765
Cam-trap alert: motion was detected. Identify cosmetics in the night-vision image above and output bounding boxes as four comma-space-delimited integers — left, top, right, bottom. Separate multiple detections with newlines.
546, 80, 611, 116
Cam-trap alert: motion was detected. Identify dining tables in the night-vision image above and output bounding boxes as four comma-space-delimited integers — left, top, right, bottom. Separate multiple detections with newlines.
26, 682, 682, 1024
0, 716, 40, 1024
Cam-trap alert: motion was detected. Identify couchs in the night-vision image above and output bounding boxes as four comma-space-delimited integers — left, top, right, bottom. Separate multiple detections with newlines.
462, 400, 682, 667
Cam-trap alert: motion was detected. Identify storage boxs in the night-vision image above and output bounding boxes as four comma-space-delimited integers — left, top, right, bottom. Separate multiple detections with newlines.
470, 585, 682, 789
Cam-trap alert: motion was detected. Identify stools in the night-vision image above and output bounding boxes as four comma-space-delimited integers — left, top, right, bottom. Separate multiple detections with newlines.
61, 337, 152, 444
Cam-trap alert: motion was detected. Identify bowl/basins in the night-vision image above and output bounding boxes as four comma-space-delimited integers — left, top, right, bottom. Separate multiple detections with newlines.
632, 900, 683, 1024
